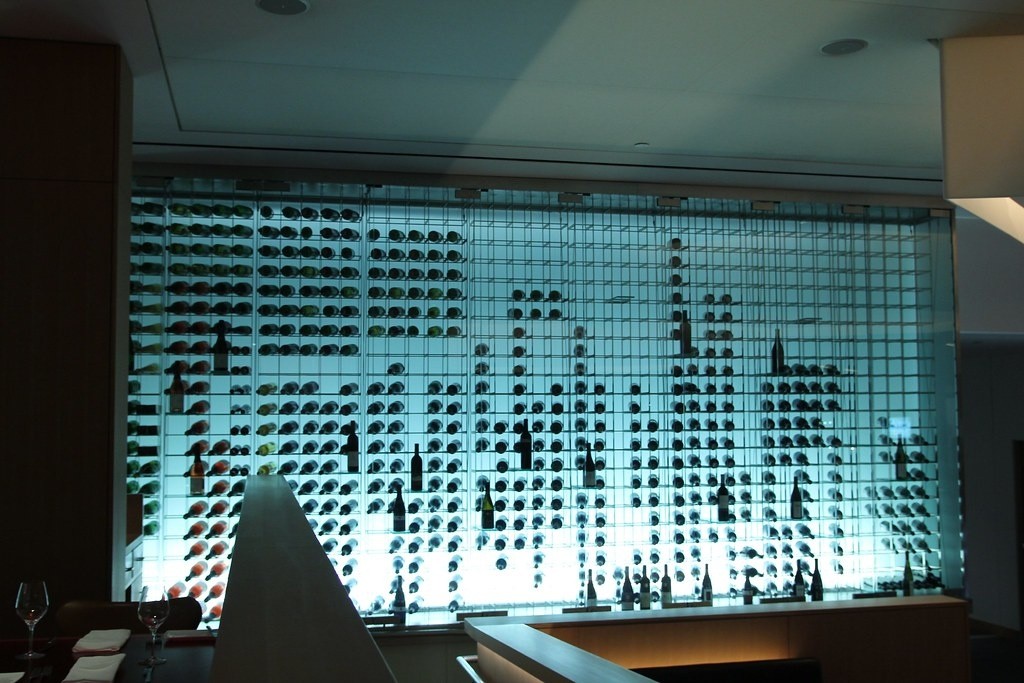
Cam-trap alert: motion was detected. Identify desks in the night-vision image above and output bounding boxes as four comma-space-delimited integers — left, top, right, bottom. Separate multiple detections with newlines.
0, 627, 220, 683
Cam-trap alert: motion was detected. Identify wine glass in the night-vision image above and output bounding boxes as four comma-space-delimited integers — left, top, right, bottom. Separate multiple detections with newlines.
15, 580, 50, 660
138, 586, 171, 668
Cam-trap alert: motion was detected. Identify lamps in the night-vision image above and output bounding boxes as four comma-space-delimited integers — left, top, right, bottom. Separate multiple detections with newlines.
250, 180, 291, 192
929, 209, 950, 218
136, 176, 170, 188
752, 200, 774, 210
657, 197, 682, 207
455, 189, 482, 199
842, 206, 864, 214
558, 192, 584, 204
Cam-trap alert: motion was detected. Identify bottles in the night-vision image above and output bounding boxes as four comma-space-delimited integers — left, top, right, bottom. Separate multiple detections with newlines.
660, 563, 672, 608
903, 550, 913, 597
612, 384, 660, 611
256, 383, 359, 596
125, 198, 165, 374
761, 328, 844, 604
257, 203, 359, 358
165, 202, 253, 374
671, 238, 684, 338
126, 380, 160, 537
864, 486, 943, 590
472, 342, 597, 613
595, 383, 606, 585
506, 288, 563, 339
673, 363, 766, 606
367, 228, 463, 339
165, 374, 250, 624
705, 293, 734, 358
368, 364, 463, 626
574, 325, 585, 338
681, 308, 692, 355
877, 417, 932, 483
692, 345, 701, 360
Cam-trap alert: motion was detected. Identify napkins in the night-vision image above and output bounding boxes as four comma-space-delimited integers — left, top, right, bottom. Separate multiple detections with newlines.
61, 654, 127, 683
73, 628, 131, 652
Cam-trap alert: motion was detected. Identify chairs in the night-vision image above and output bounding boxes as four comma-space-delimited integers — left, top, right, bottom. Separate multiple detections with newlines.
53, 597, 202, 636
